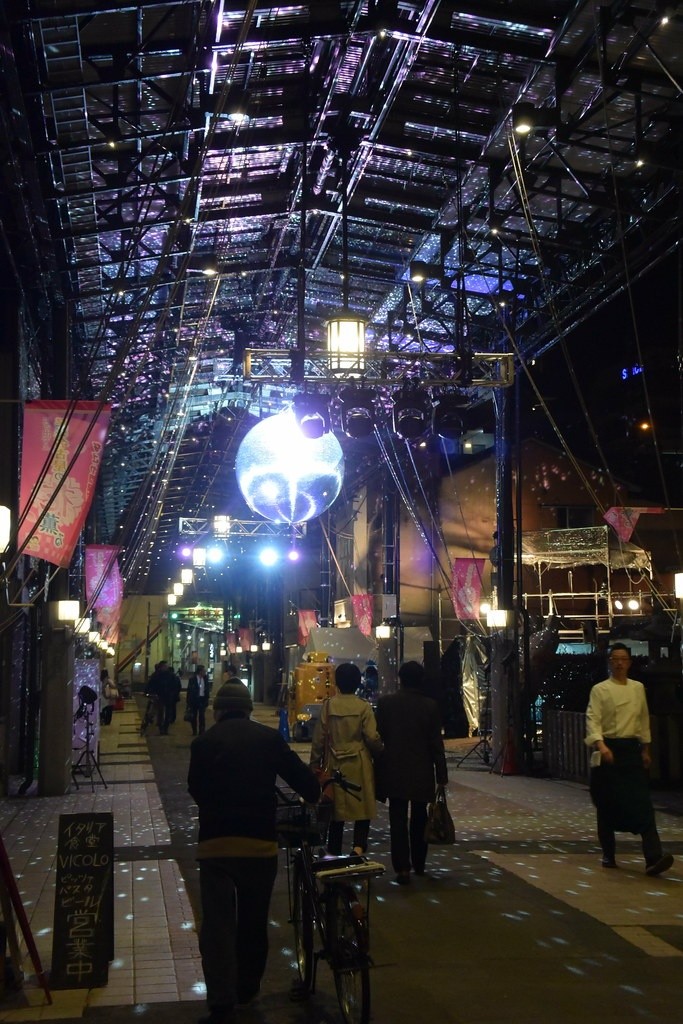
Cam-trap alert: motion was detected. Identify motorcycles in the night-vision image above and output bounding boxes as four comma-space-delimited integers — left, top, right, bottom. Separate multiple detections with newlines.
118, 681, 132, 700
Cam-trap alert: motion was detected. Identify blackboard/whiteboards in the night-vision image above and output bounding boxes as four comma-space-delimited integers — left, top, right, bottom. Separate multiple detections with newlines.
50, 812, 115, 991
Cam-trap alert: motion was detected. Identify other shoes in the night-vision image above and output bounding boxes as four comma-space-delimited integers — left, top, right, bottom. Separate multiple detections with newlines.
192, 729, 197, 735
160, 725, 169, 735
237, 998, 252, 1006
394, 871, 411, 885
350, 850, 357, 856
646, 854, 674, 876
601, 853, 616, 865
413, 863, 424, 875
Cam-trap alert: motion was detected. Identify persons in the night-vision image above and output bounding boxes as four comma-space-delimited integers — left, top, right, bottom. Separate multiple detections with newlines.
585, 642, 674, 877
100, 669, 117, 725
187, 678, 319, 1024
309, 664, 384, 856
144, 661, 182, 736
375, 661, 448, 885
188, 665, 209, 736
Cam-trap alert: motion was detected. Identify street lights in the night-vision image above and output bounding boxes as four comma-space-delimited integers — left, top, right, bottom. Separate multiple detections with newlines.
376, 618, 404, 666
486, 605, 533, 769
220, 634, 280, 699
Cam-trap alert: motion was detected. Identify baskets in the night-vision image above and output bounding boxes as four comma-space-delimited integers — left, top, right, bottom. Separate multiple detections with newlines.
275, 792, 331, 849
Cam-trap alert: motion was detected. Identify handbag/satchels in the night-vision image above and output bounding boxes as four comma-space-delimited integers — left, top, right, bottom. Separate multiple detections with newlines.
423, 786, 455, 844
313, 768, 334, 801
184, 705, 194, 721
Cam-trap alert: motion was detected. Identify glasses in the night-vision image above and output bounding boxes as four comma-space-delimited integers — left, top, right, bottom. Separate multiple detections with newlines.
609, 656, 630, 663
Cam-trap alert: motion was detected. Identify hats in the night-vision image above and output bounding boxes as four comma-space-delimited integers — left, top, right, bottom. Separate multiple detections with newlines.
213, 677, 253, 710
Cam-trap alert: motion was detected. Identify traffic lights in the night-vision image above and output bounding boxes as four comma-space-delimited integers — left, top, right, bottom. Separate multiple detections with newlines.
170, 612, 184, 618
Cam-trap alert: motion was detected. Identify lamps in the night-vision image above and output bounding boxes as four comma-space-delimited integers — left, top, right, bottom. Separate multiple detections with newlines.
88, 632, 100, 643
58, 601, 80, 620
74, 618, 90, 634
376, 625, 391, 639
324, 132, 366, 377
220, 643, 271, 658
390, 389, 431, 438
97, 641, 115, 657
487, 610, 508, 627
291, 394, 331, 439
338, 388, 376, 439
429, 394, 471, 440
167, 441, 232, 606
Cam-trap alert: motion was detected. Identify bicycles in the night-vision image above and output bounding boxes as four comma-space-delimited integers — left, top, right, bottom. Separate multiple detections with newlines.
138, 694, 158, 736
274, 776, 382, 1024
